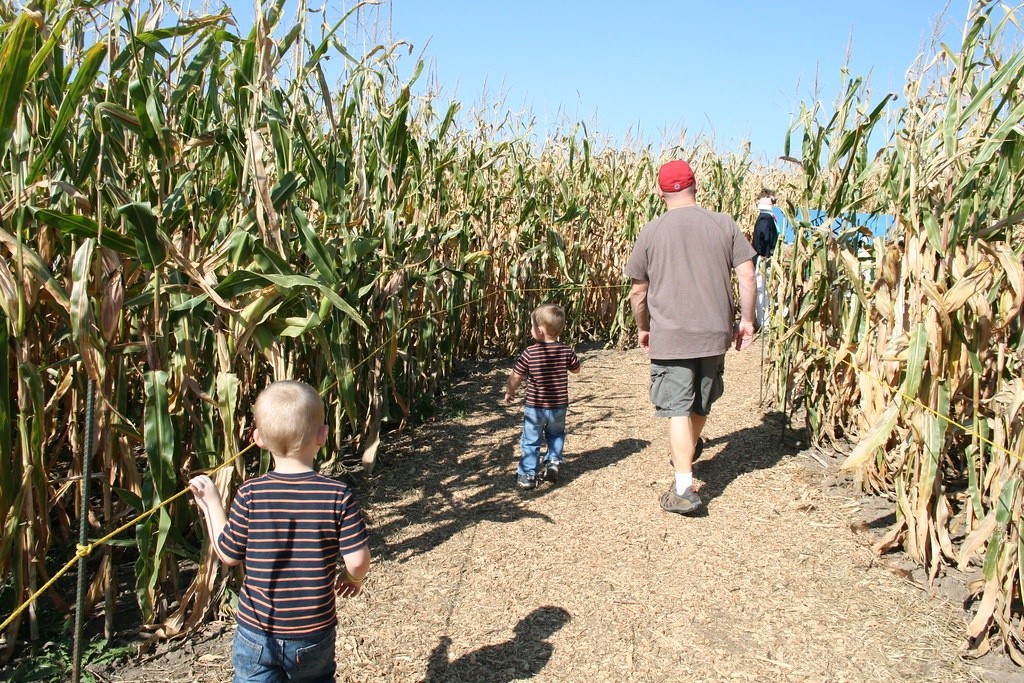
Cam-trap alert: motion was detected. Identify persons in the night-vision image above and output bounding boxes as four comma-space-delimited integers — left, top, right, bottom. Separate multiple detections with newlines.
504, 303, 580, 489
623, 160, 757, 513
752, 189, 777, 333
190, 379, 370, 683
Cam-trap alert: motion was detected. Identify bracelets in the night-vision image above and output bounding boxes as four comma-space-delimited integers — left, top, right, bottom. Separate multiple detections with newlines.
347, 570, 364, 583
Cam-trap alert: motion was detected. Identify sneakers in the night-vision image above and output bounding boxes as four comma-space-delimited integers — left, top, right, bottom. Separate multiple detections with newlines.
670, 437, 703, 467
660, 479, 702, 514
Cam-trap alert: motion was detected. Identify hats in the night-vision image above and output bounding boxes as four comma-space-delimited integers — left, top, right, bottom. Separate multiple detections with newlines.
659, 160, 694, 192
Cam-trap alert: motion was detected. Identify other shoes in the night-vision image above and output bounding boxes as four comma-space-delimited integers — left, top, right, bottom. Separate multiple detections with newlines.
517, 474, 535, 489
541, 469, 558, 481
